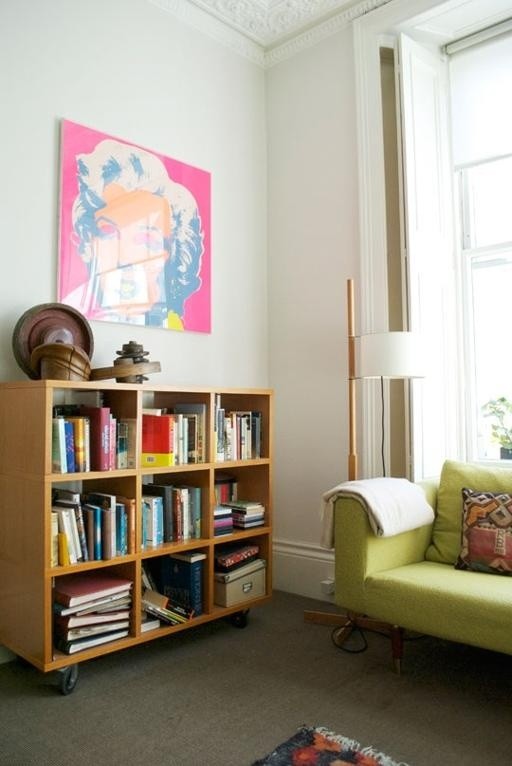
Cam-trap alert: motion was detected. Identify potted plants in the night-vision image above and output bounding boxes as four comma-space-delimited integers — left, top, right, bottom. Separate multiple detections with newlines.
482, 396, 512, 459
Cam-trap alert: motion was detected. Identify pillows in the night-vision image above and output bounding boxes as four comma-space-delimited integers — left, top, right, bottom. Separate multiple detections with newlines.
425, 459, 512, 565
455, 488, 512, 576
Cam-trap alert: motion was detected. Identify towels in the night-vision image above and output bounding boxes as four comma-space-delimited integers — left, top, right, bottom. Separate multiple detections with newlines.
319, 479, 436, 550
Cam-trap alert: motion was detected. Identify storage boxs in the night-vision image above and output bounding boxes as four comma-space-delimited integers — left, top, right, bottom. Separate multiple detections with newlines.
214, 559, 267, 608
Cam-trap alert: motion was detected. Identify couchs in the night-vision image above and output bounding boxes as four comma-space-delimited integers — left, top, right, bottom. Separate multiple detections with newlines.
332, 459, 512, 674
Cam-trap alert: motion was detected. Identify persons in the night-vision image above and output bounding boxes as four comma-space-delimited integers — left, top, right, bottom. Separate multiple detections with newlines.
58, 139, 205, 330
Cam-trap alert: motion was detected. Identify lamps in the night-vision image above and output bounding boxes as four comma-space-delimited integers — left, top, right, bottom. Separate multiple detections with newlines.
303, 278, 429, 643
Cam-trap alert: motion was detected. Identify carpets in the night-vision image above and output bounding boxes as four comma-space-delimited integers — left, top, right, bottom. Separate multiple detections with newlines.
250, 724, 408, 765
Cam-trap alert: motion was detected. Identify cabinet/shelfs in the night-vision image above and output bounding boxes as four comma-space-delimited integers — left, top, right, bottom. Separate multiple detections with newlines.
0, 379, 273, 694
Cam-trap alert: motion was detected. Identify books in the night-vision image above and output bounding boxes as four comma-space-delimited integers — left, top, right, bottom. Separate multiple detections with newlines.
140, 551, 208, 633
51, 570, 133, 655
213, 473, 266, 537
50, 487, 136, 569
142, 483, 201, 551
217, 408, 262, 462
140, 402, 207, 468
52, 390, 130, 475
